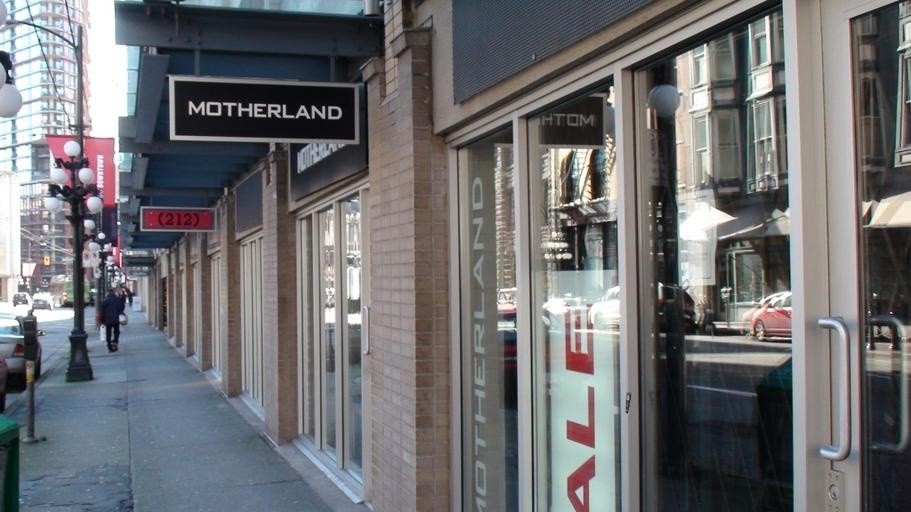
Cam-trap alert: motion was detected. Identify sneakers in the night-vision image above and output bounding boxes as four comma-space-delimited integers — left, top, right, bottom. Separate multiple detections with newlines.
108, 342, 117, 352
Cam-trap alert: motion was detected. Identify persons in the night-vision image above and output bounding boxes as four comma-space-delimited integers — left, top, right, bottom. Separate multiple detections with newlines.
96, 288, 124, 354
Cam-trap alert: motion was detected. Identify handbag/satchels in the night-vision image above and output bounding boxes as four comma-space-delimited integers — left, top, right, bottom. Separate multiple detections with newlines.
119, 314, 128, 324
100, 324, 115, 341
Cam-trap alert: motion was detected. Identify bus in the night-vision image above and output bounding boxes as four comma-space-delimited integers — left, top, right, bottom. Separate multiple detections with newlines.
60, 279, 92, 307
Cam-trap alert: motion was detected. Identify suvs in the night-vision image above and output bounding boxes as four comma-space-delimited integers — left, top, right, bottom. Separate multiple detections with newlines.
589, 282, 696, 333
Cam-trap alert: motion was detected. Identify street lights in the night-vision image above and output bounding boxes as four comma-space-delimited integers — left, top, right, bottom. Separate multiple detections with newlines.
50, 139, 104, 384
0, 17, 86, 354
91, 230, 119, 328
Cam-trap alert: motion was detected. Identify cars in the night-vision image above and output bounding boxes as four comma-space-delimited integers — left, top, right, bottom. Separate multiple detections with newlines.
749, 289, 793, 340
13, 290, 54, 310
543, 294, 592, 330
497, 322, 517, 414
496, 286, 518, 319
2, 314, 43, 413
